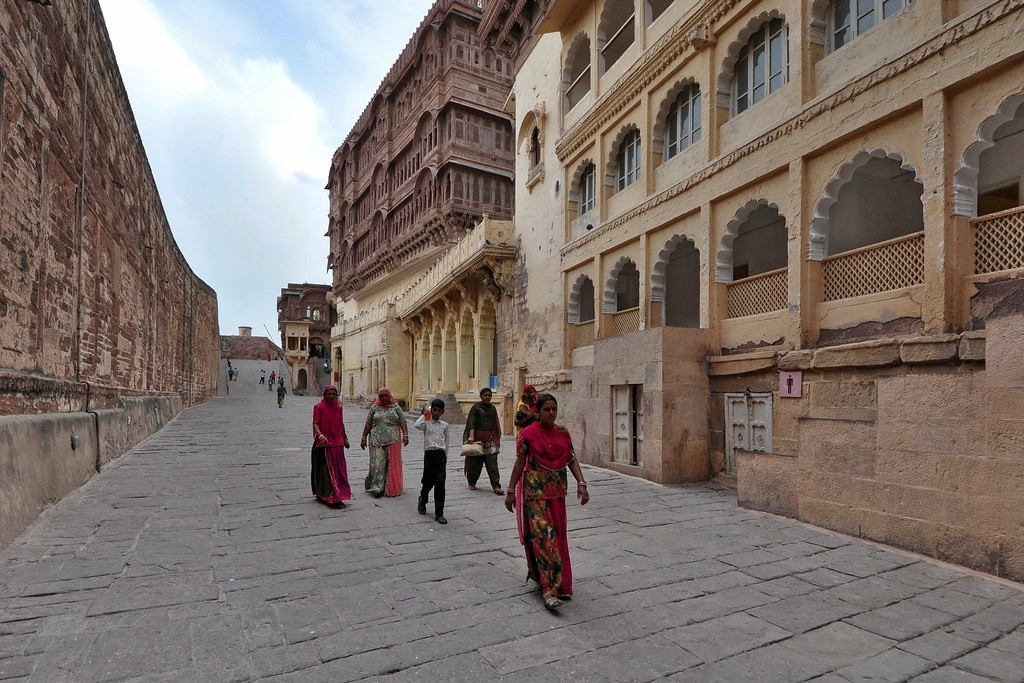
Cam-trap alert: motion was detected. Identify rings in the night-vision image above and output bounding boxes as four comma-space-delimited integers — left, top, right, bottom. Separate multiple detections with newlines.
507, 506, 509, 508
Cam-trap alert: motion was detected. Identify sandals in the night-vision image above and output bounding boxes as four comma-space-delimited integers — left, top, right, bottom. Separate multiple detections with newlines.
542, 591, 563, 609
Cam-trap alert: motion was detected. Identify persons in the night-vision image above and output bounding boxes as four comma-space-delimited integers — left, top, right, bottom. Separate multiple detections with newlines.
414, 398, 449, 524
514, 385, 541, 443
463, 388, 504, 494
227, 357, 238, 380
311, 385, 352, 508
277, 377, 287, 408
268, 371, 276, 391
259, 370, 266, 384
505, 394, 590, 609
361, 387, 409, 498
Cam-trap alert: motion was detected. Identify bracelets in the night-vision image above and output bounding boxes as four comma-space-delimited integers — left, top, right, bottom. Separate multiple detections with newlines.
403, 435, 408, 436
318, 434, 324, 438
345, 438, 348, 441
469, 433, 474, 437
362, 437, 367, 440
506, 488, 516, 494
578, 481, 587, 487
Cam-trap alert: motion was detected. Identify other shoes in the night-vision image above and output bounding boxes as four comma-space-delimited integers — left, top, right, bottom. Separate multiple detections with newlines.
434, 516, 448, 524
469, 485, 476, 489
332, 500, 345, 509
493, 486, 505, 495
417, 497, 426, 514
316, 495, 323, 504
